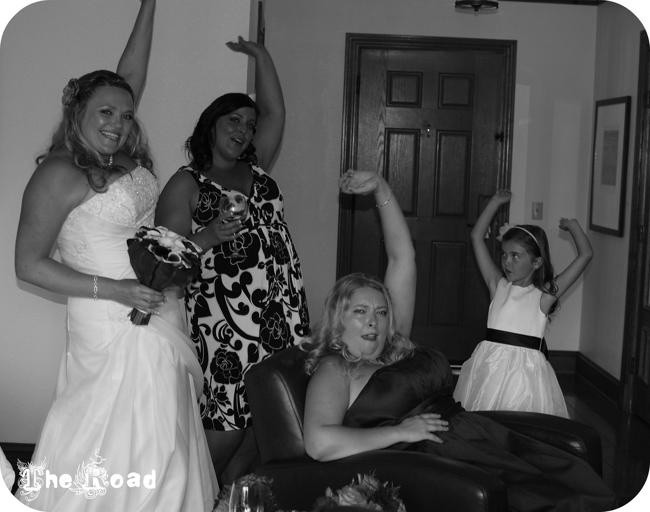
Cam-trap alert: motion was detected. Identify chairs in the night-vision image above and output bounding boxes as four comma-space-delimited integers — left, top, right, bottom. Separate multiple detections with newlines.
244, 341, 604, 511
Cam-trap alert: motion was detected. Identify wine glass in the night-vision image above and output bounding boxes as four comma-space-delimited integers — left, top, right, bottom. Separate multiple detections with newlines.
219, 196, 249, 259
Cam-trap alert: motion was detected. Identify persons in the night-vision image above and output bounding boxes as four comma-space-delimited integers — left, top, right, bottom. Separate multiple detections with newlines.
303, 169, 615, 512
15, 0, 221, 512
154, 35, 315, 478
451, 187, 593, 422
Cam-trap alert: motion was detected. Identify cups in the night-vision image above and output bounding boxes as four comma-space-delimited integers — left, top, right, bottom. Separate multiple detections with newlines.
228, 478, 263, 512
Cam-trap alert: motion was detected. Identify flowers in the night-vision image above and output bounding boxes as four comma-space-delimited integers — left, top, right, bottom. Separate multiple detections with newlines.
126, 224, 205, 326
310, 468, 408, 512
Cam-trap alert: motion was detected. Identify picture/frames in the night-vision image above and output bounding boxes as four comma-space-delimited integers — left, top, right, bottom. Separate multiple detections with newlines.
589, 95, 633, 238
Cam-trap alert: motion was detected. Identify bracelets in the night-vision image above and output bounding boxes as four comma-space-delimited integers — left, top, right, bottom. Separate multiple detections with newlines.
92, 274, 99, 300
374, 190, 394, 208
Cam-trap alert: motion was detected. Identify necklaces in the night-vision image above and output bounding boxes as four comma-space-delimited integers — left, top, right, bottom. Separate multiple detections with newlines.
101, 154, 113, 167
